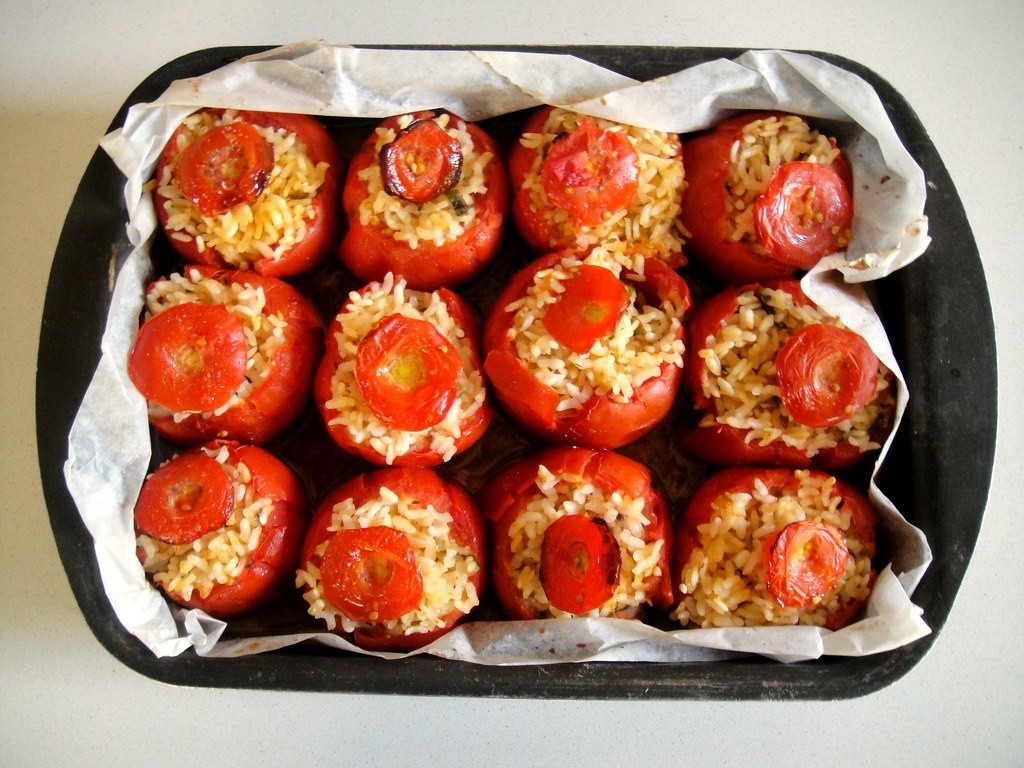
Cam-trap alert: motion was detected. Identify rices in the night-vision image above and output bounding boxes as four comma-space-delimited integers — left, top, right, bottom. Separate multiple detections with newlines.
135, 107, 892, 626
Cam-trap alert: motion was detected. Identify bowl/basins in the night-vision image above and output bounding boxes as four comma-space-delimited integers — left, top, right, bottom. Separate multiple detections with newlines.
35, 46, 1000, 703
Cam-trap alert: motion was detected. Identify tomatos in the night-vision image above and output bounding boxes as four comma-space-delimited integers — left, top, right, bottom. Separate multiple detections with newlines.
131, 105, 892, 651
